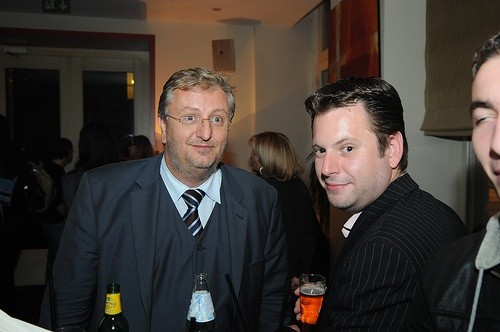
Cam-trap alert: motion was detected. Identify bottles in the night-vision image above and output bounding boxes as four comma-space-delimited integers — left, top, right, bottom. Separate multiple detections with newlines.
97, 283, 130, 332
185, 273, 216, 332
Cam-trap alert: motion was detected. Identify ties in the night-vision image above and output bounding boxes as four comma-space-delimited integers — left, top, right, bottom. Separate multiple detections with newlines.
179, 188, 204, 238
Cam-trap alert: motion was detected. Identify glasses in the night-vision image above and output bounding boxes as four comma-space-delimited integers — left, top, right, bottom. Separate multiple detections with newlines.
163, 114, 232, 130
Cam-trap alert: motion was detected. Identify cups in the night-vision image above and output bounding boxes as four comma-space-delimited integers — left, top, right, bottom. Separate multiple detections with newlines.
298, 273, 327, 331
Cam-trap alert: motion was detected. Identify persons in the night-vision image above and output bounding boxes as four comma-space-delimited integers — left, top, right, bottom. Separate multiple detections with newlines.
275, 77, 467, 332
125, 134, 153, 159
40, 67, 294, 332
60, 122, 123, 225
398, 31, 500, 332
248, 131, 329, 281
0, 114, 74, 332
